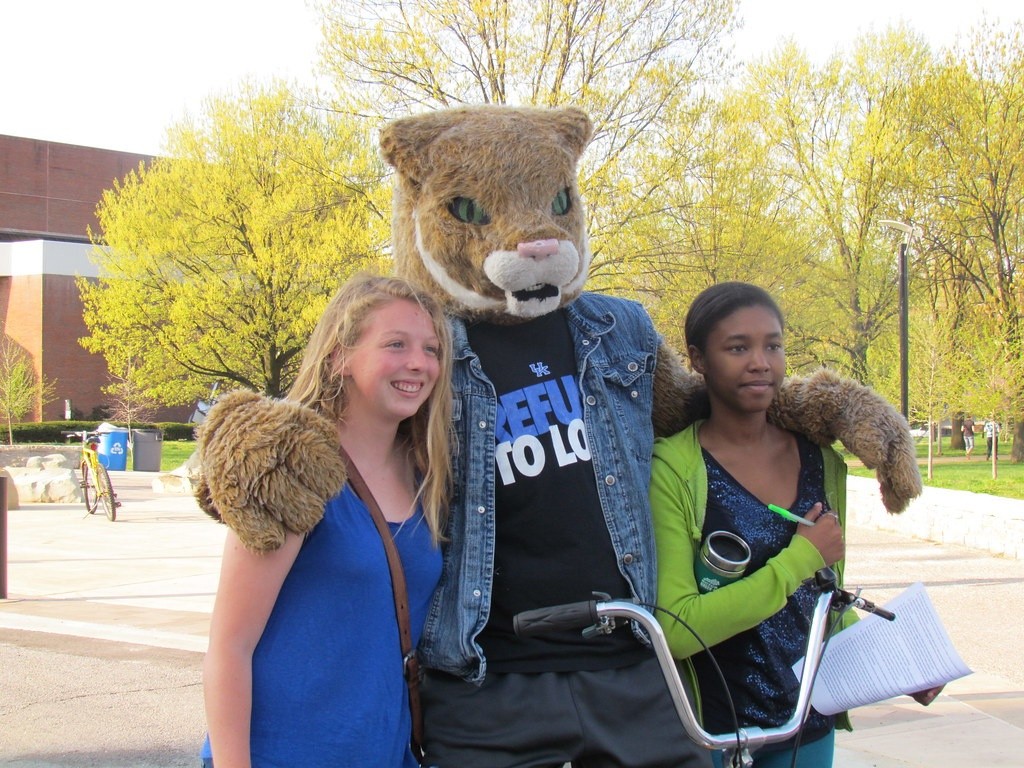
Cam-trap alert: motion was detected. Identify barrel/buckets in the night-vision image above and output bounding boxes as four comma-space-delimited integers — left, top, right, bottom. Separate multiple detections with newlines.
129, 429, 164, 473
98, 431, 128, 470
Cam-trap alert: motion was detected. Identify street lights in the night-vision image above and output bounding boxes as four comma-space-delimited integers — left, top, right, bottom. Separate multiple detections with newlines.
878, 219, 922, 423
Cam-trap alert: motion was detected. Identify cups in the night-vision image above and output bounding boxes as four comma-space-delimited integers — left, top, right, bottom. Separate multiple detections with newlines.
693, 530, 751, 594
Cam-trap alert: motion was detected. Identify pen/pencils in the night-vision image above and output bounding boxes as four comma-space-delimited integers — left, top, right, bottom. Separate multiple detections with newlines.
766, 502, 816, 529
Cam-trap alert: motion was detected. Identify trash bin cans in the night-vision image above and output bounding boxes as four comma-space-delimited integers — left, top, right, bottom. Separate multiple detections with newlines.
131, 428, 160, 473
97, 430, 128, 471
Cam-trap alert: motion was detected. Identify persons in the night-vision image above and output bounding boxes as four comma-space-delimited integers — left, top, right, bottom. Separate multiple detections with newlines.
203, 276, 458, 768
646, 283, 947, 768
983, 420, 1000, 462
961, 416, 975, 460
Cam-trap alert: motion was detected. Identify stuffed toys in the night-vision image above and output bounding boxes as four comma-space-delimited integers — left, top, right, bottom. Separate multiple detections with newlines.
186, 108, 924, 768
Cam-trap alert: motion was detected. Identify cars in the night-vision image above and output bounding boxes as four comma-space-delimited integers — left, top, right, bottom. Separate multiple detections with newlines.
909, 420, 931, 439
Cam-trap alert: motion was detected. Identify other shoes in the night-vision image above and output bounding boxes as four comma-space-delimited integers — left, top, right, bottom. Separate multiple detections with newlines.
965, 452, 970, 459
985, 458, 989, 460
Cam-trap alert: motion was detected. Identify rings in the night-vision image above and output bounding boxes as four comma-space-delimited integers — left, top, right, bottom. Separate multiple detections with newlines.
829, 512, 839, 522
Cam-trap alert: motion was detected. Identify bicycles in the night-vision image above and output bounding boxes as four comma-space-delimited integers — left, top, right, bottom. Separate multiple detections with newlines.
509, 564, 898, 768
66, 429, 121, 521
